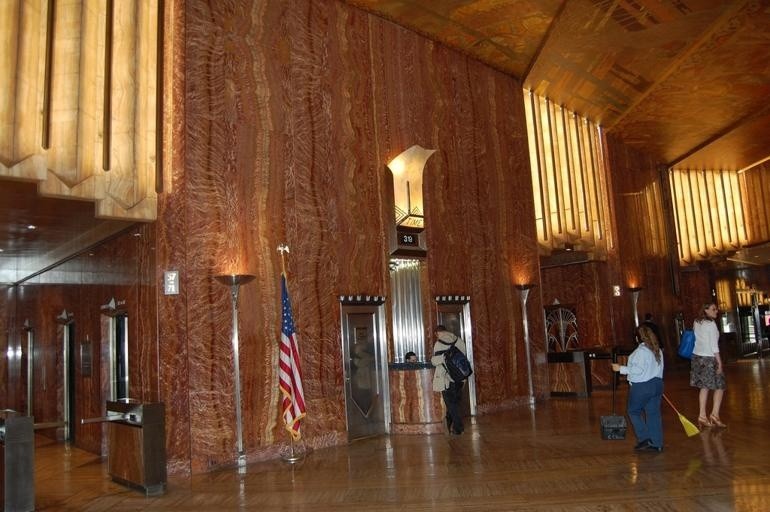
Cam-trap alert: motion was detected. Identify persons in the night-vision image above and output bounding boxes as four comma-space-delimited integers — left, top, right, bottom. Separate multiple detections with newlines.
611, 326, 665, 452
688, 302, 726, 429
405, 350, 417, 362
430, 324, 467, 436
640, 313, 661, 342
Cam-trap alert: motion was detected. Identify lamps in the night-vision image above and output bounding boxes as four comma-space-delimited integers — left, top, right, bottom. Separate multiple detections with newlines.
387, 145, 438, 233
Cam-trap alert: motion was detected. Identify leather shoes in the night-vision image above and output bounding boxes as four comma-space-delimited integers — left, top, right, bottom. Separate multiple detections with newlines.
634, 438, 664, 453
443, 415, 464, 435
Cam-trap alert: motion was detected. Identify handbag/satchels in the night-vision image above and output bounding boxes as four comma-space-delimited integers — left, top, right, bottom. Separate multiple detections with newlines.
678, 330, 695, 358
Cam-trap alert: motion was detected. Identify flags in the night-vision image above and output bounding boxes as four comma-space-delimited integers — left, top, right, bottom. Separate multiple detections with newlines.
277, 273, 307, 440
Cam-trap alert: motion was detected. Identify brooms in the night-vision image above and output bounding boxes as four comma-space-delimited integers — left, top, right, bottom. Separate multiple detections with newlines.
661, 393, 700, 438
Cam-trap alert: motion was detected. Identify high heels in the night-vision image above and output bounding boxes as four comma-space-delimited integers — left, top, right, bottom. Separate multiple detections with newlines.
698, 413, 727, 430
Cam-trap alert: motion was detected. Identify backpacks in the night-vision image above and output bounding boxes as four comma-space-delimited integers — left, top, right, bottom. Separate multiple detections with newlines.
435, 335, 473, 383
